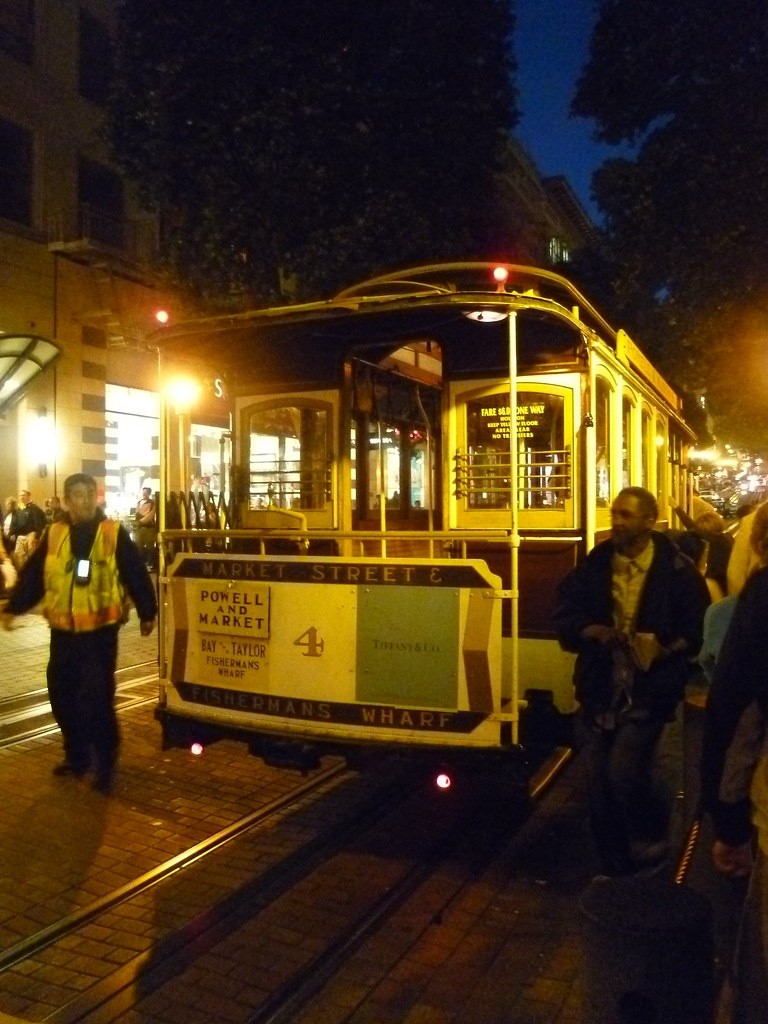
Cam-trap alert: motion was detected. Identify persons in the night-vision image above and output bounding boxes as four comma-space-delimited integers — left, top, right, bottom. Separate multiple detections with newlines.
2, 474, 160, 785
553, 487, 710, 889
669, 487, 767, 874
0, 487, 155, 597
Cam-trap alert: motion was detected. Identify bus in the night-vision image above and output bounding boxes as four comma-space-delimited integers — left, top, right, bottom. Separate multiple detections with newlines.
144, 263, 716, 790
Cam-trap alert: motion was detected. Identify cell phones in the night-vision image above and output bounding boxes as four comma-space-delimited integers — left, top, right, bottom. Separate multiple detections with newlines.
76, 557, 92, 582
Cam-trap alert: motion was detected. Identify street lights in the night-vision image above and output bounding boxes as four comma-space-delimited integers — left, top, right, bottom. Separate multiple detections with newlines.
164, 373, 201, 495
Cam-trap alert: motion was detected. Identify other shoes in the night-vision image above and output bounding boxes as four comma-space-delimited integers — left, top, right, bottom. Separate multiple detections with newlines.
93, 768, 112, 790
52, 757, 89, 776
628, 833, 666, 868
